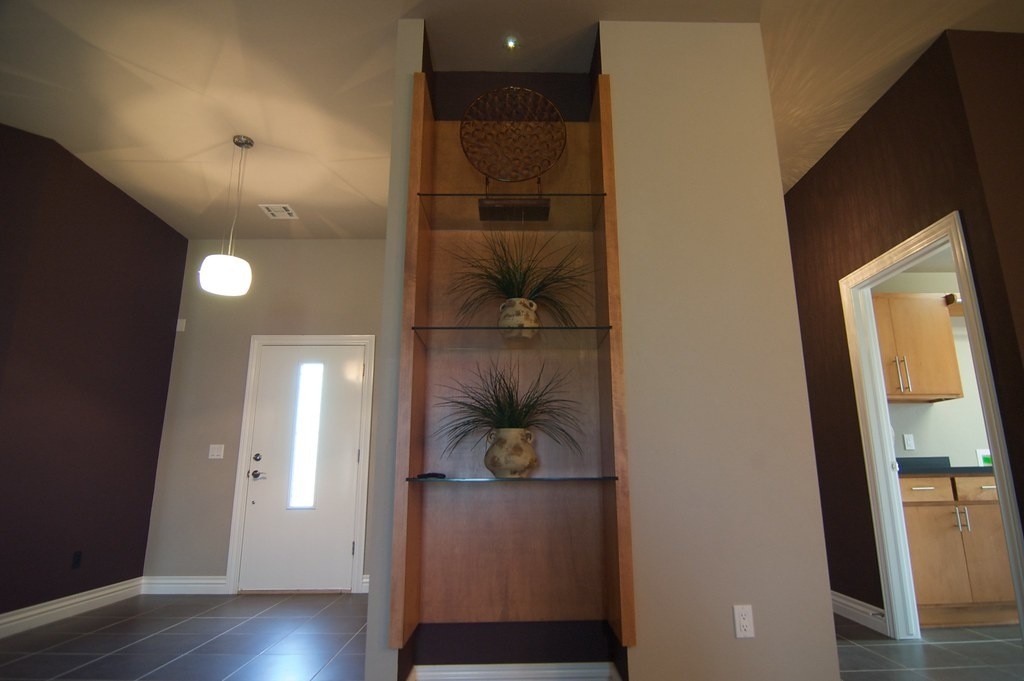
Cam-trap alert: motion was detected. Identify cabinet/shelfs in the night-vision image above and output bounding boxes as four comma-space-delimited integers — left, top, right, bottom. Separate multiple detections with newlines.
388, 64, 640, 650
872, 293, 964, 404
900, 474, 1019, 627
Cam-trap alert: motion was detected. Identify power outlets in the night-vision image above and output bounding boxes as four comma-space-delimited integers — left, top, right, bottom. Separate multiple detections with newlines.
903, 433, 915, 450
733, 605, 755, 639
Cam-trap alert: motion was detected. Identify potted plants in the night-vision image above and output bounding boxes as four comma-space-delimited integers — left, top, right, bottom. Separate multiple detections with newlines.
443, 217, 599, 342
430, 350, 586, 478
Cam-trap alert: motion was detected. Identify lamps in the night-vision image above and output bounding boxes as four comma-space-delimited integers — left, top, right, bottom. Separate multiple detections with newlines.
198, 135, 255, 296
